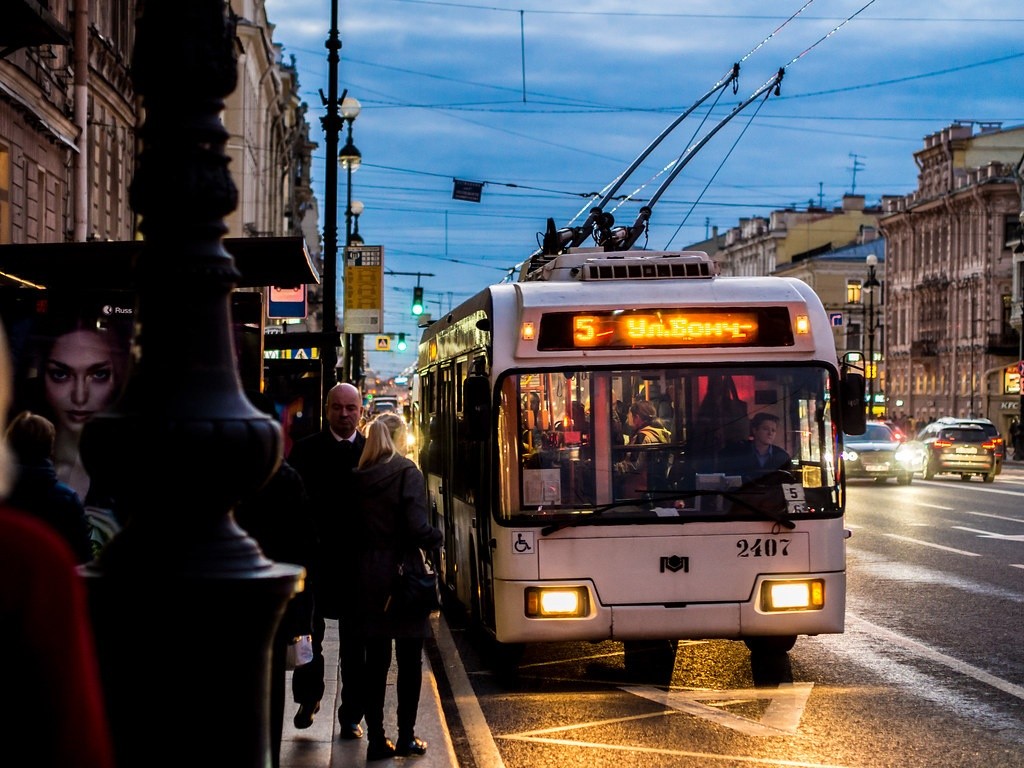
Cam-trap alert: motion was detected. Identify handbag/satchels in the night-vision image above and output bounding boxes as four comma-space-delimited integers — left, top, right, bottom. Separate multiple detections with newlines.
394, 569, 439, 611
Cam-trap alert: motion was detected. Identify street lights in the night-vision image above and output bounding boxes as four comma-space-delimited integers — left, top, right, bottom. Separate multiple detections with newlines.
861, 253, 883, 419
336, 93, 366, 386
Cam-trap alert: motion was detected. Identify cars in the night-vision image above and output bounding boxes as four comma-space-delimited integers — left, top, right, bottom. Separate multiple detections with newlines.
842, 422, 914, 485
884, 421, 907, 444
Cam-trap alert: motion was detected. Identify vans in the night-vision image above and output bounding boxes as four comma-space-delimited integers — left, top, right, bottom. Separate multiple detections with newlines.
374, 394, 397, 416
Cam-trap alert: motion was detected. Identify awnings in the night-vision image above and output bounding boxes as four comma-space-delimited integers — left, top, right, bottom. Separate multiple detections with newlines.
0, 234, 322, 299
263, 331, 342, 351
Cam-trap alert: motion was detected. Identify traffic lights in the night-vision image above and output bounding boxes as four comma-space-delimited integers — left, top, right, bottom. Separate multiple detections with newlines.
411, 286, 424, 316
398, 333, 406, 350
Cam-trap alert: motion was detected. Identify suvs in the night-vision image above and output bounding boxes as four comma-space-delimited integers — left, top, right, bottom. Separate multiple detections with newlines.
938, 415, 1005, 474
916, 421, 996, 482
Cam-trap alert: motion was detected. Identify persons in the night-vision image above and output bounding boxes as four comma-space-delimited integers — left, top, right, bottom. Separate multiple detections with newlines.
15, 289, 139, 508
715, 412, 792, 489
293, 383, 367, 747
869, 411, 937, 437
352, 409, 443, 764
242, 394, 309, 768
7, 411, 98, 566
520, 392, 672, 506
429, 421, 442, 474
1009, 415, 1024, 460
0, 315, 115, 768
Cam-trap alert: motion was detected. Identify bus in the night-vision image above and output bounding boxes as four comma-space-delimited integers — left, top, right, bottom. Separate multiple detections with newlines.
416, 61, 869, 670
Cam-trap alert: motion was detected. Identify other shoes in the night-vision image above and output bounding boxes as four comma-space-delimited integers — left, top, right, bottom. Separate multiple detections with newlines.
394, 736, 429, 758
294, 700, 320, 729
341, 722, 361, 739
366, 739, 396, 761
